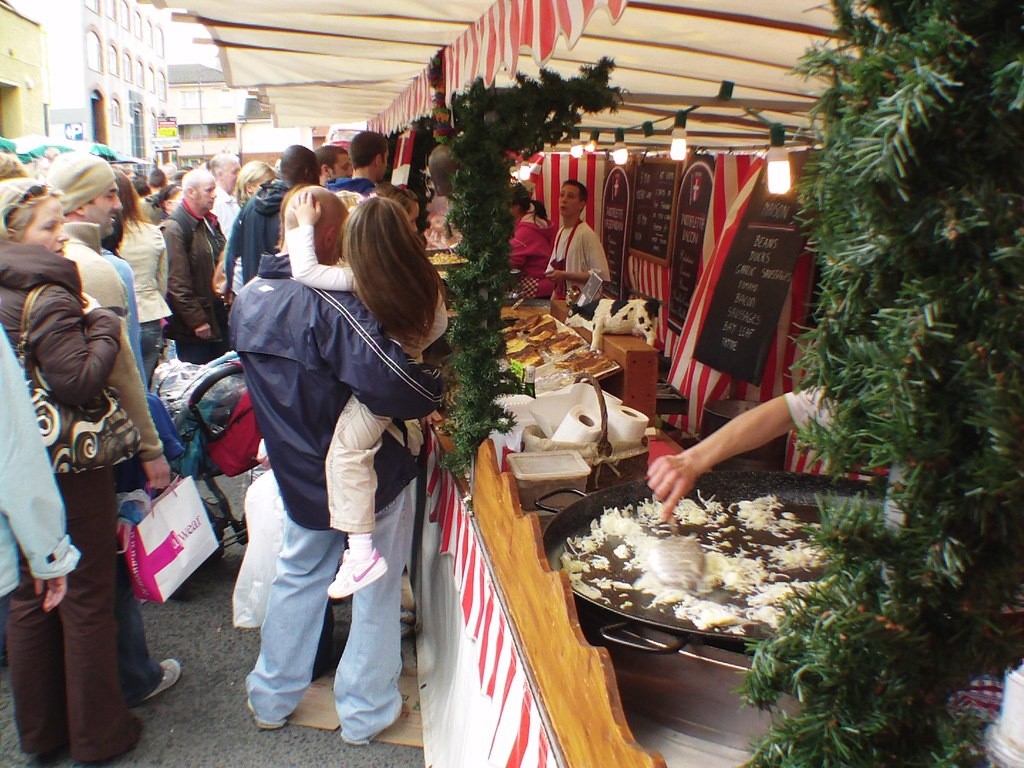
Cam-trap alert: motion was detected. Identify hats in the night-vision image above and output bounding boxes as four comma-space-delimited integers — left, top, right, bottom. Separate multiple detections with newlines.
43, 146, 114, 215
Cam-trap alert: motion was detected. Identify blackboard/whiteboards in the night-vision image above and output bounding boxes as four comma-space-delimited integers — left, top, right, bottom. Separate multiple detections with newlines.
666, 153, 718, 337
628, 157, 682, 267
690, 149, 810, 387
599, 167, 632, 302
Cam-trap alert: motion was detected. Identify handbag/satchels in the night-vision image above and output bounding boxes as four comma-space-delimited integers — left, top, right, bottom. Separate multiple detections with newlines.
16, 284, 143, 474
162, 286, 224, 345
232, 467, 287, 629
118, 473, 219, 605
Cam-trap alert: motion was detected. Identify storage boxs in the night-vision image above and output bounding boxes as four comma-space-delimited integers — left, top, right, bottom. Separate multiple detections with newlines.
505, 449, 592, 512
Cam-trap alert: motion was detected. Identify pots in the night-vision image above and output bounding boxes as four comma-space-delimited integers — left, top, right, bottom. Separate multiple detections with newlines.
534, 472, 876, 655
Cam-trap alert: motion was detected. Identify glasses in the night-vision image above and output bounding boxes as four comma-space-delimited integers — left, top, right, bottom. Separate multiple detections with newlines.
3, 185, 48, 227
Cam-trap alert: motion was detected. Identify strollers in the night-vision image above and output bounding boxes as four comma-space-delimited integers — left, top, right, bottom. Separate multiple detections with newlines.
126, 349, 262, 566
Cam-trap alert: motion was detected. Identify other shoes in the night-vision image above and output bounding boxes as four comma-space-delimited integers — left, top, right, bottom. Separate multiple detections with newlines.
247, 696, 282, 729
341, 689, 403, 744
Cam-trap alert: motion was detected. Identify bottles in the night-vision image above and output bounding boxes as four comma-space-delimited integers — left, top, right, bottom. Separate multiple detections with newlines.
523, 367, 536, 398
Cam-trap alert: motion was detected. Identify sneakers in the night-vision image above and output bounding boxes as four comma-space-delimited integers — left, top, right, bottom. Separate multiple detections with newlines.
326, 547, 388, 598
143, 659, 181, 701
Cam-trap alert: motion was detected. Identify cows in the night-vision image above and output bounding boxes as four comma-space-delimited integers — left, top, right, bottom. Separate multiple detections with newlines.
564, 297, 660, 351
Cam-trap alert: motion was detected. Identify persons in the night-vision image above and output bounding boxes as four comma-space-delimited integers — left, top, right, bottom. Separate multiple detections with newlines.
228, 186, 450, 745
47, 150, 181, 707
0, 177, 143, 761
0, 131, 420, 669
509, 184, 555, 301
285, 192, 448, 597
647, 383, 912, 588
543, 179, 610, 320
